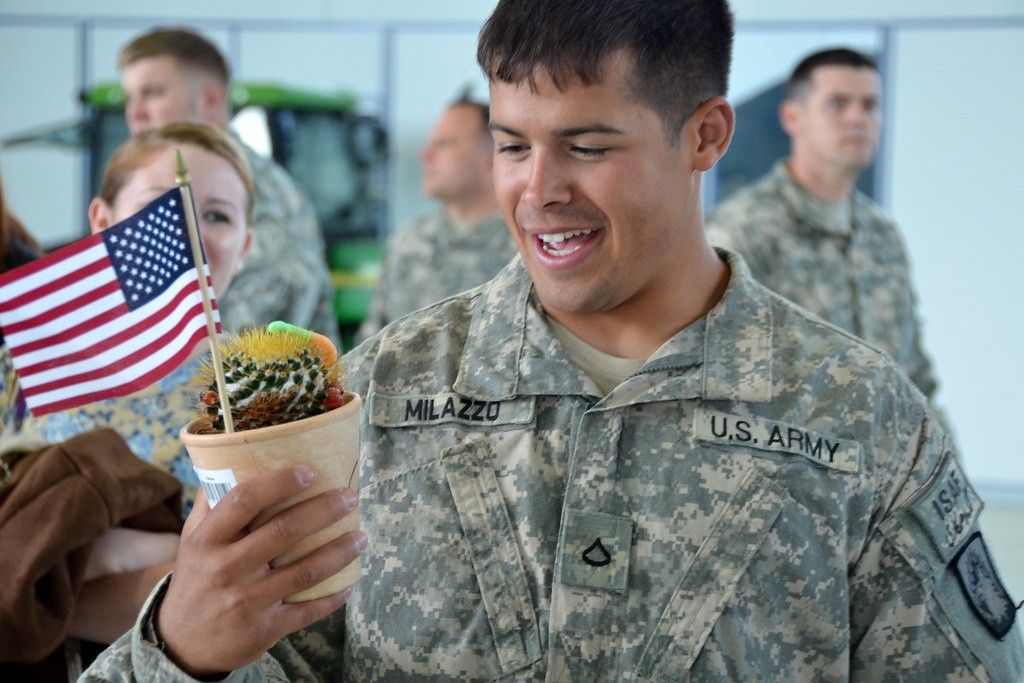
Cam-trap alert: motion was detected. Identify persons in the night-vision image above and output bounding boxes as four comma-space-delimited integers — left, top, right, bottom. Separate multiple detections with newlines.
354, 89, 528, 352
704, 46, 938, 432
1, 119, 265, 681
118, 24, 347, 383
76, 0, 1024, 683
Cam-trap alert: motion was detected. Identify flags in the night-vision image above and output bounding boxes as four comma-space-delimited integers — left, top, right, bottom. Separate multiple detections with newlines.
0, 186, 224, 421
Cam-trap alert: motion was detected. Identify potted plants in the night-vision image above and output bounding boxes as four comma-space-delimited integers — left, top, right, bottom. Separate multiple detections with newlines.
1, 143, 364, 602
